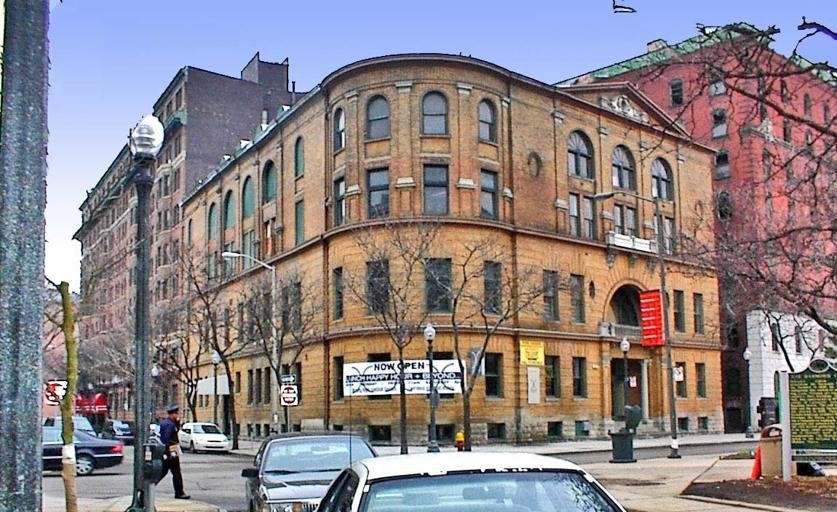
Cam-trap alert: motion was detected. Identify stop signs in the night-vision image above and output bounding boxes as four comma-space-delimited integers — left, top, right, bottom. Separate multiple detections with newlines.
280, 386, 299, 406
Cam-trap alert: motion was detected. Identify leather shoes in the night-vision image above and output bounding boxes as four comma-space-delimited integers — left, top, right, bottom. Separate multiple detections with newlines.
180, 494, 189, 498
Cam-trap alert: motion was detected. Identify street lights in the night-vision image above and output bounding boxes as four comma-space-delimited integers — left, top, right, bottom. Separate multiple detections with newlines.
125, 112, 161, 508
618, 335, 634, 431
592, 190, 681, 459
208, 352, 220, 426
151, 364, 158, 422
740, 347, 756, 439
422, 321, 440, 453
222, 249, 279, 435
86, 381, 93, 408
113, 374, 120, 419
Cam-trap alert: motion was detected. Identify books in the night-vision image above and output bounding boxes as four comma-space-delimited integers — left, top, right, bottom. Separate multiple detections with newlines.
167, 444, 184, 457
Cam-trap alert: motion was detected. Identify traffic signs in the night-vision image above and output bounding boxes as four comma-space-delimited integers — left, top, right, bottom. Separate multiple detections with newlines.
279, 373, 296, 384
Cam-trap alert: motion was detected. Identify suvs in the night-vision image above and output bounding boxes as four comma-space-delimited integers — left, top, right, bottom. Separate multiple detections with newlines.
100, 418, 134, 442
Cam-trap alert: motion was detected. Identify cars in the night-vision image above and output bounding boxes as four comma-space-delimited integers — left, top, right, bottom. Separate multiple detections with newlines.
38, 426, 122, 479
176, 422, 229, 452
315, 452, 624, 512
241, 431, 379, 510
149, 423, 161, 435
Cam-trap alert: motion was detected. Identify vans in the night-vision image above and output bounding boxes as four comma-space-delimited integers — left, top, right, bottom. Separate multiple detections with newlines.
45, 416, 97, 439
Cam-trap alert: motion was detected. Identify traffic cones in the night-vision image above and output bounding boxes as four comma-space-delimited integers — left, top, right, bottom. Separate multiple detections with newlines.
750, 445, 761, 482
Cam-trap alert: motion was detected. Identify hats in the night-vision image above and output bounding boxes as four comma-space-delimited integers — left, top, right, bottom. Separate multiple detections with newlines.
167, 405, 178, 413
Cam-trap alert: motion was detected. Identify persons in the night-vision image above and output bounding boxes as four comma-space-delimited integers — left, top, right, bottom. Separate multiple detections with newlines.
150, 404, 191, 498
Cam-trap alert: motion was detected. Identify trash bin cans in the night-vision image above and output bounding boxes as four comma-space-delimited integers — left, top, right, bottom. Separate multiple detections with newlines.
760, 424, 783, 478
609, 405, 642, 462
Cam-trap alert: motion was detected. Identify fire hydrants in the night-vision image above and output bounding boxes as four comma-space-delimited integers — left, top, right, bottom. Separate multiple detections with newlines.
454, 430, 466, 452
